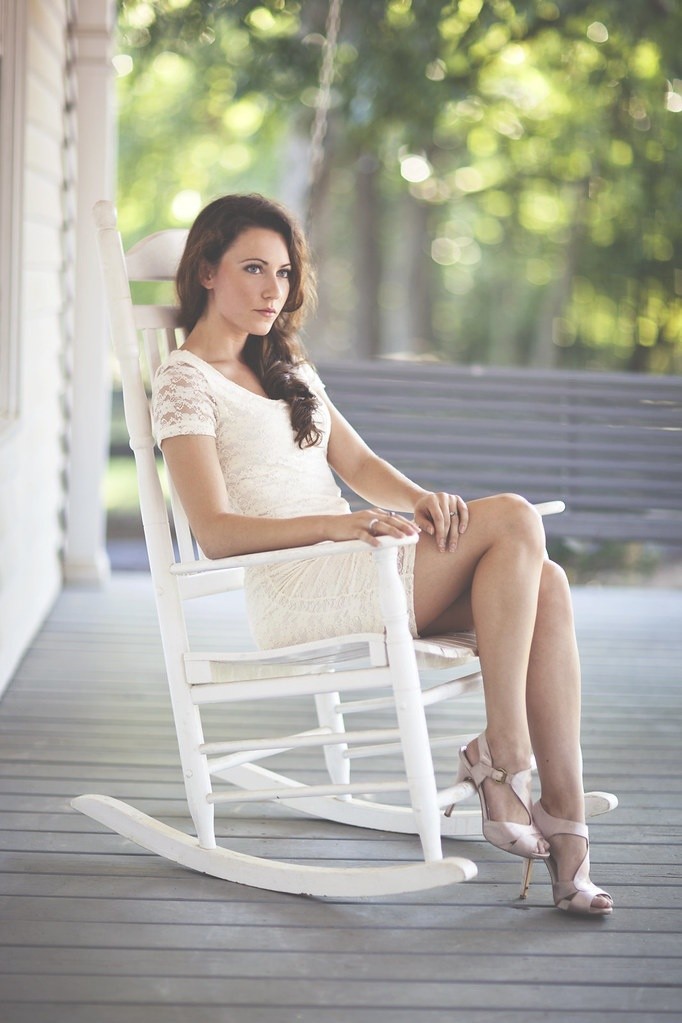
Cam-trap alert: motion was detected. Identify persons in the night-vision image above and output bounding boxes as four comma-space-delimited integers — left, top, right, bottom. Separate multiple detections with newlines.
149, 194, 614, 915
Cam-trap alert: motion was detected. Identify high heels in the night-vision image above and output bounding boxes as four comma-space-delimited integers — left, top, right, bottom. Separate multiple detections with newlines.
444, 731, 550, 858
520, 792, 619, 914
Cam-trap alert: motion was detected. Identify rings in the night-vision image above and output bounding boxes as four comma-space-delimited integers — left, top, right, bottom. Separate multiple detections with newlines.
450, 513, 456, 516
390, 512, 395, 517
369, 519, 378, 528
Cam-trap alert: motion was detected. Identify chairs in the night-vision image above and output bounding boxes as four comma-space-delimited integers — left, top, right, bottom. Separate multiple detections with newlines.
73, 201, 618, 897
